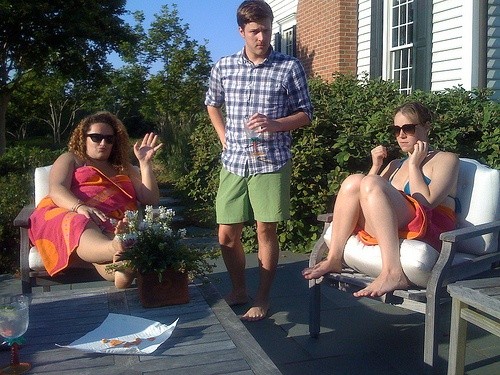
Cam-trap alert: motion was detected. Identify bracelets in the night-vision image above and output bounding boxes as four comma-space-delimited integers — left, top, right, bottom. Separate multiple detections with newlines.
71, 199, 86, 213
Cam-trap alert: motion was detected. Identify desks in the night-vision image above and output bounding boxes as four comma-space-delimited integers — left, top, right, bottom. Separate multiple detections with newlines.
445, 276, 500, 375
0, 285, 284, 375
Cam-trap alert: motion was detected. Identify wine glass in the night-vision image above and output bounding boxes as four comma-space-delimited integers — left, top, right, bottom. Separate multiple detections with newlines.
243, 116, 266, 157
0, 295, 32, 375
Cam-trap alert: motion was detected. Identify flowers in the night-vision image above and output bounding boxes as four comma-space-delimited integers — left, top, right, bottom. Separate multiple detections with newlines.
104, 204, 222, 290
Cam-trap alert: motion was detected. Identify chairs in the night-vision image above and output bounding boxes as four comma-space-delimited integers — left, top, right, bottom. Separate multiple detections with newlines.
305, 158, 500, 375
12, 164, 143, 294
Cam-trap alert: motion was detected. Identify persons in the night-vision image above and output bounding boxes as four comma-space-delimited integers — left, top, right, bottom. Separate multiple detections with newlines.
205, 0, 313, 321
302, 101, 460, 298
27, 110, 163, 289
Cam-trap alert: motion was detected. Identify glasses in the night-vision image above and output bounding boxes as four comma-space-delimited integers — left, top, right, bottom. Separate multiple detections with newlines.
85, 133, 116, 144
390, 123, 425, 138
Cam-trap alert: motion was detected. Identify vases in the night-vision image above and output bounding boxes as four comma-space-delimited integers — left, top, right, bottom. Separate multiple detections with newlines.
134, 270, 191, 310
